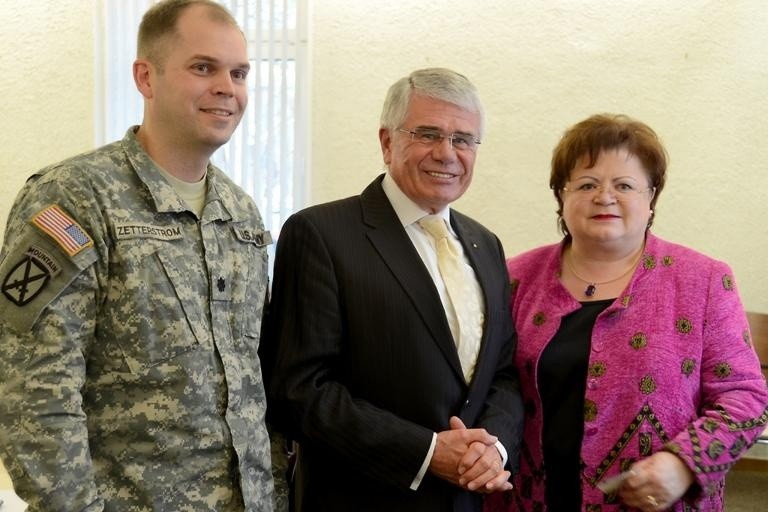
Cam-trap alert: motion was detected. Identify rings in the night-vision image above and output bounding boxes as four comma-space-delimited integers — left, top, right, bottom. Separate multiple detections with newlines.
646, 493, 659, 507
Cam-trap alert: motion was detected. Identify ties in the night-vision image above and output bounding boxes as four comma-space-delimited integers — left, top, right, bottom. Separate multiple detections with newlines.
419, 215, 482, 382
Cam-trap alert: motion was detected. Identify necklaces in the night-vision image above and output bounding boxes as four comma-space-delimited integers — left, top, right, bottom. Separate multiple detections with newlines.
568, 241, 645, 297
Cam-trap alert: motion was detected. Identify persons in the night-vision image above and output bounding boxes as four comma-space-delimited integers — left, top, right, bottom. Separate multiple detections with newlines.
254, 63, 530, 510
3, 0, 292, 509
472, 108, 768, 512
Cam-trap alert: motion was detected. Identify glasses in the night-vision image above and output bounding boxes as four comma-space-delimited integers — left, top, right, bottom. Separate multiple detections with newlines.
399, 128, 480, 149
563, 182, 650, 199
